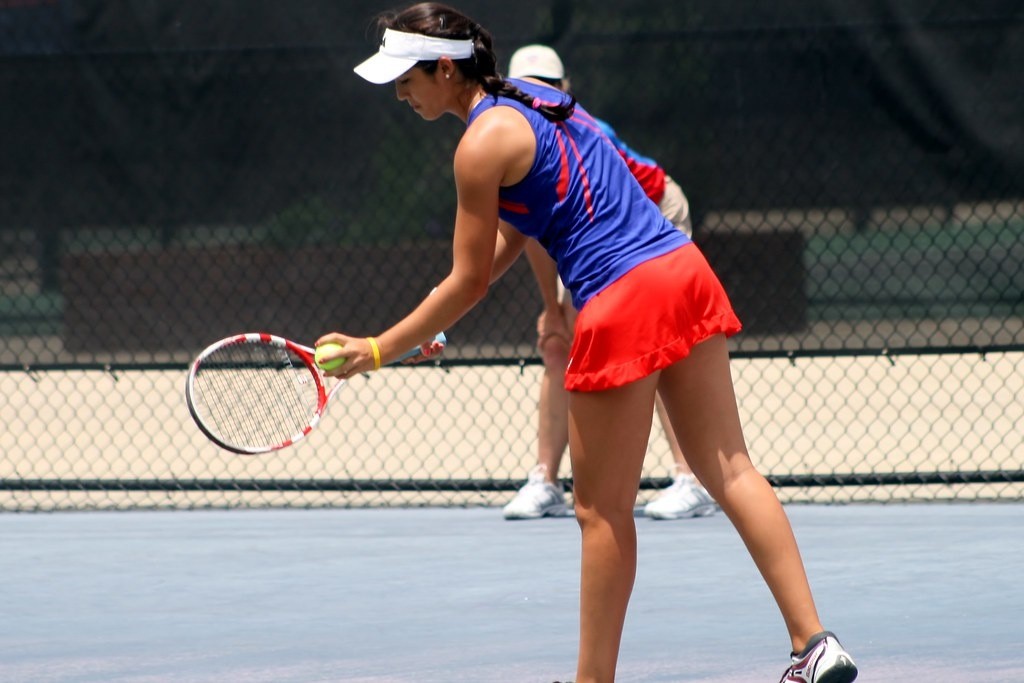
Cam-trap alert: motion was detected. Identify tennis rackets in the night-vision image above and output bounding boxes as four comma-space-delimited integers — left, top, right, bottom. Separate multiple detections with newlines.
185, 330, 448, 457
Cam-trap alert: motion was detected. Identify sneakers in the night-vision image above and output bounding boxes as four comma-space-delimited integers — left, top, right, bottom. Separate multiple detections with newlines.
644, 473, 717, 520
780, 631, 858, 683
502, 473, 567, 520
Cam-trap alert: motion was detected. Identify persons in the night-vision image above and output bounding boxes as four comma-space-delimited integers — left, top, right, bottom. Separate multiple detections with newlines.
313, 0, 862, 683
497, 43, 716, 519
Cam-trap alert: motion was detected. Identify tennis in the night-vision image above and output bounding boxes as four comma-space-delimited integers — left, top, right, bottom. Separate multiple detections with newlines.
314, 342, 346, 371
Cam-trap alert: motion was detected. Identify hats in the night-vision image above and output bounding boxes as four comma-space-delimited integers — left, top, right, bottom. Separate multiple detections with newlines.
508, 46, 563, 79
353, 27, 474, 84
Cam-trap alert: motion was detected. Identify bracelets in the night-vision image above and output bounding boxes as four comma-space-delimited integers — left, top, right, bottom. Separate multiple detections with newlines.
366, 336, 380, 371
